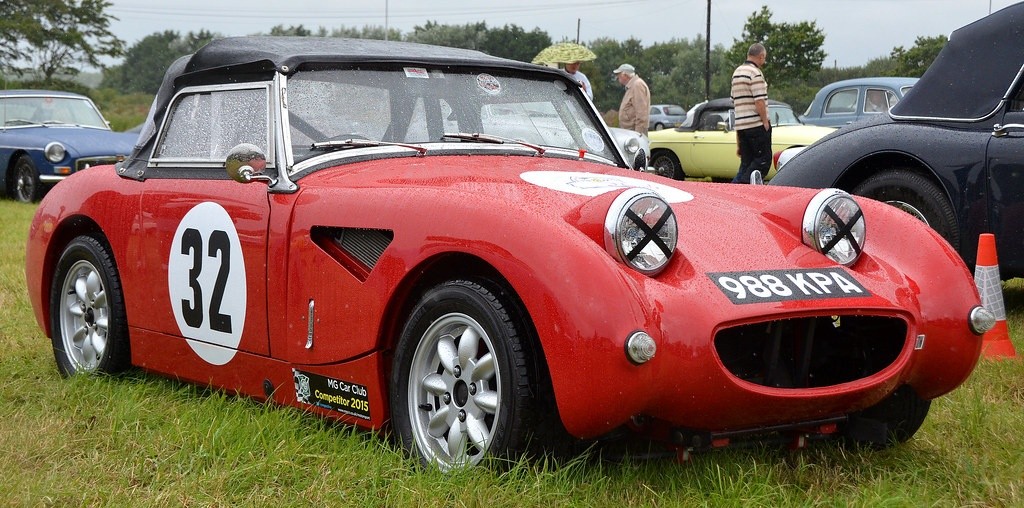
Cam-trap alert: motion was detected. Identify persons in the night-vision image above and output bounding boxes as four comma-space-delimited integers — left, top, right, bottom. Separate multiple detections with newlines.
730, 42, 772, 184
866, 90, 887, 112
736, 131, 742, 158
558, 61, 594, 101
613, 64, 651, 138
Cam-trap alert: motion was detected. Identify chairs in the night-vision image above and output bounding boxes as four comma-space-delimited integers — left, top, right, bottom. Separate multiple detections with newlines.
31, 107, 53, 121
705, 114, 724, 131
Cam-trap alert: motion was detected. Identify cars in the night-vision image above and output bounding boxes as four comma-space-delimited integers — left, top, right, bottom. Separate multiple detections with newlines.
647, 104, 688, 132
751, 1, 1024, 283
0, 89, 141, 206
480, 99, 652, 173
25, 36, 998, 479
796, 77, 920, 131
648, 96, 840, 183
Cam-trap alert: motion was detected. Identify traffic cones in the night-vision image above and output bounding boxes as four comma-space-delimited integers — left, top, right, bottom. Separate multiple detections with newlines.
974, 234, 1021, 361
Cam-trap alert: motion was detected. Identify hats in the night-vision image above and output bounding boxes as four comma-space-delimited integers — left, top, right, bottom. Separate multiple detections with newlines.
613, 64, 635, 74
